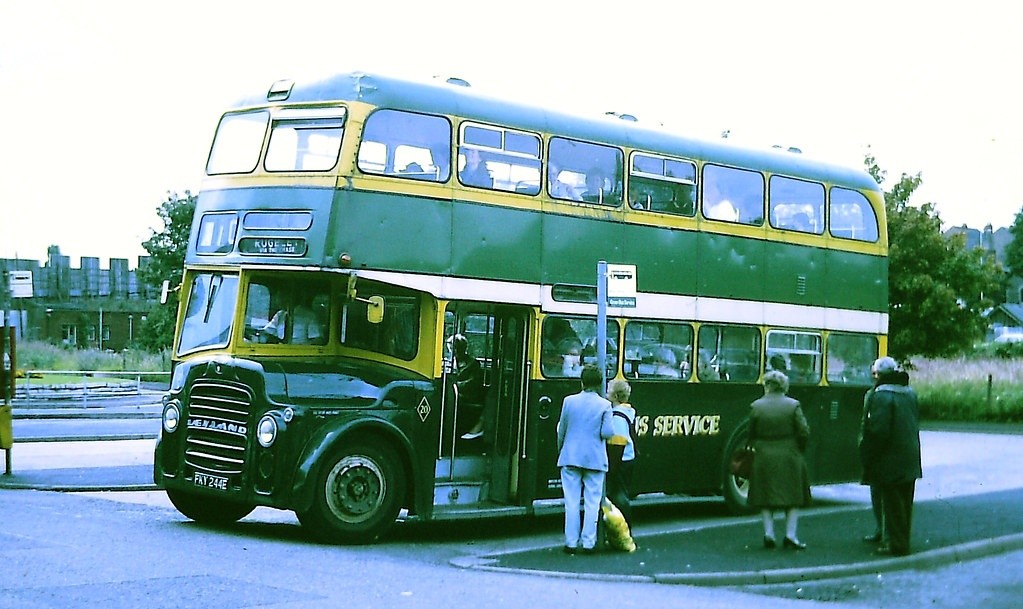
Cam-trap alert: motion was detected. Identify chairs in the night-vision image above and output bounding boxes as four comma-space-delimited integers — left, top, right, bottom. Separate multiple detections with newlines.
275, 162, 868, 416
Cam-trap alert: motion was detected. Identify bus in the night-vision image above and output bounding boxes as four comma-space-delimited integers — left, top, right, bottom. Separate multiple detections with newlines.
152, 70, 895, 551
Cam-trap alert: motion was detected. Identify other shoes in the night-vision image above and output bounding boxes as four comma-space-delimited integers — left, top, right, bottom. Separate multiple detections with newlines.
863, 527, 881, 543
461, 429, 484, 439
876, 540, 910, 556
563, 545, 576, 554
583, 546, 596, 553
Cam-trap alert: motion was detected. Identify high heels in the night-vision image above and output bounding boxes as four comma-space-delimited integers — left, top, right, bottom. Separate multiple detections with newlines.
783, 535, 807, 549
764, 536, 775, 547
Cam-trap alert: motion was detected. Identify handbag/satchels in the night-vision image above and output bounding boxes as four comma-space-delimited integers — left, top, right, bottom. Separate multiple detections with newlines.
600, 497, 638, 553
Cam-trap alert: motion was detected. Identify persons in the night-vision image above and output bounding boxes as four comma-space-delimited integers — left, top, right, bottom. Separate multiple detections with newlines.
599, 374, 637, 547
861, 359, 889, 546
429, 143, 456, 181
579, 168, 620, 206
628, 188, 645, 210
261, 279, 324, 352
676, 342, 704, 383
857, 357, 926, 560
787, 213, 811, 236
442, 333, 488, 427
789, 349, 820, 389
768, 350, 793, 387
557, 364, 614, 556
460, 146, 496, 188
536, 158, 587, 203
743, 368, 813, 549
